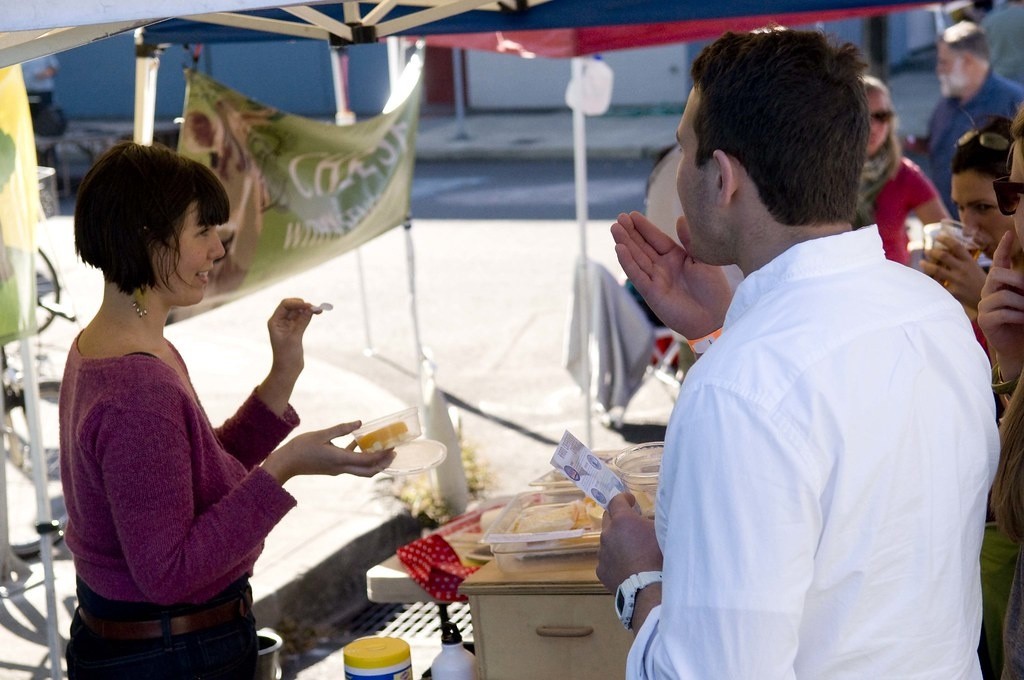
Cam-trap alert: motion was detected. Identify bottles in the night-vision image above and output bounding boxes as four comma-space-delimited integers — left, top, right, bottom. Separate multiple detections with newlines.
563, 53, 615, 114
344, 636, 413, 680
431, 622, 475, 680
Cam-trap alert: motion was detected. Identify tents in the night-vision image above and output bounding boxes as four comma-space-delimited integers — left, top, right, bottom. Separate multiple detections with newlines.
378, 2, 948, 389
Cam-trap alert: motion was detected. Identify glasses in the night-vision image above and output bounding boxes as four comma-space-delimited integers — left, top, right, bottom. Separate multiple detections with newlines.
991, 175, 1024, 216
870, 109, 895, 124
953, 127, 1010, 152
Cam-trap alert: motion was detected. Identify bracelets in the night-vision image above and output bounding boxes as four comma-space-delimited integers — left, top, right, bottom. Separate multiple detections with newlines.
686, 327, 723, 354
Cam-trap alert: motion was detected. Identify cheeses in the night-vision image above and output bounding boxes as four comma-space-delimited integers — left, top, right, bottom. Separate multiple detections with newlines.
358, 422, 409, 452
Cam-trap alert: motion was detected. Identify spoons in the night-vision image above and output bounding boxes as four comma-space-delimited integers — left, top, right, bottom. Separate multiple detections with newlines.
310, 303, 332, 310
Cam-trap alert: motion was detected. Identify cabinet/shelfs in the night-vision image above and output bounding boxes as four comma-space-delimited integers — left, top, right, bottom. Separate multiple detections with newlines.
458, 560, 635, 680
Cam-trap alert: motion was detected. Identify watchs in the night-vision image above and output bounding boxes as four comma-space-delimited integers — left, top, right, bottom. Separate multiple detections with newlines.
614, 570, 663, 633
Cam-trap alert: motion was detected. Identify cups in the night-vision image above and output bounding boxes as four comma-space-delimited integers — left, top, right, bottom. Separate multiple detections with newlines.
926, 218, 992, 298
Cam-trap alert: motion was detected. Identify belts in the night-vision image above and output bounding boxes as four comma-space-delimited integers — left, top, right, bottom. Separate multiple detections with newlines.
76, 587, 253, 641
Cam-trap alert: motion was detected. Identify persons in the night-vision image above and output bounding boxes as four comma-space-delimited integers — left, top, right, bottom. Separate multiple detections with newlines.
595, 31, 1000, 680
856, 0, 1024, 680
59, 143, 396, 680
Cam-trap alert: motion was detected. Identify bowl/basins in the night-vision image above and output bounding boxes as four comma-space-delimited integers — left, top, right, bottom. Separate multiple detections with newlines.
613, 441, 664, 513
352, 407, 422, 453
